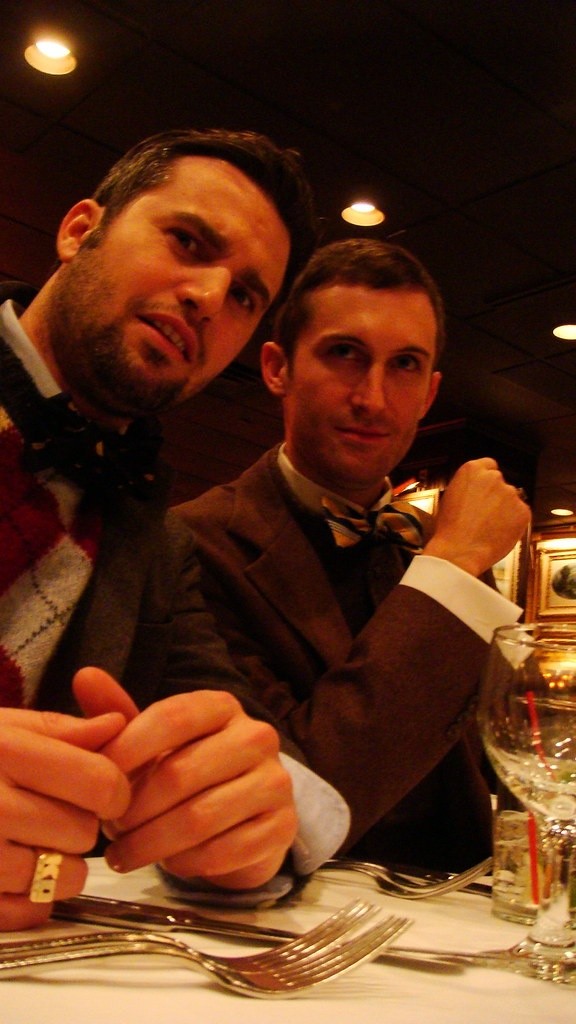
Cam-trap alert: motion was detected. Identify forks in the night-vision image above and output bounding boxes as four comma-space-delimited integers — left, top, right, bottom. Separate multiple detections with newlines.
319, 856, 496, 900
0, 914, 415, 1000
0, 898, 384, 974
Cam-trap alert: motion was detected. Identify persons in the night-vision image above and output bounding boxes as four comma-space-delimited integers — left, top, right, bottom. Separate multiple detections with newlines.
166, 238, 531, 880
0, 127, 299, 932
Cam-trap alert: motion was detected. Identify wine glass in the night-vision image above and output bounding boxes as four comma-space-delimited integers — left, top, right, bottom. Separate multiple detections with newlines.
477, 622, 576, 991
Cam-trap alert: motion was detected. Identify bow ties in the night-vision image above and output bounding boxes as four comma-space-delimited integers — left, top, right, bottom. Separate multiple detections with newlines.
322, 494, 424, 554
0, 339, 164, 502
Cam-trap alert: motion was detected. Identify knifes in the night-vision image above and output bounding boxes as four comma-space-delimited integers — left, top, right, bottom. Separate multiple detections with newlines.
51, 895, 507, 965
390, 864, 492, 897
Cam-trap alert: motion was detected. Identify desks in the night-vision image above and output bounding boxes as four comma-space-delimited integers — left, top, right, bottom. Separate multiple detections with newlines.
0, 841, 576, 1024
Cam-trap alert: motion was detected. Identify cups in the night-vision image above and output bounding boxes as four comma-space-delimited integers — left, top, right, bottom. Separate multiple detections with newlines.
490, 816, 539, 925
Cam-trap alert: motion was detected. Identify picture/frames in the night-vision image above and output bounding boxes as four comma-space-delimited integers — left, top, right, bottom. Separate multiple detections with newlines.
534, 550, 576, 620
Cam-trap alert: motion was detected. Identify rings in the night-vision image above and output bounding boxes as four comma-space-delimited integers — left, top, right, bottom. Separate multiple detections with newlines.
30, 849, 62, 904
